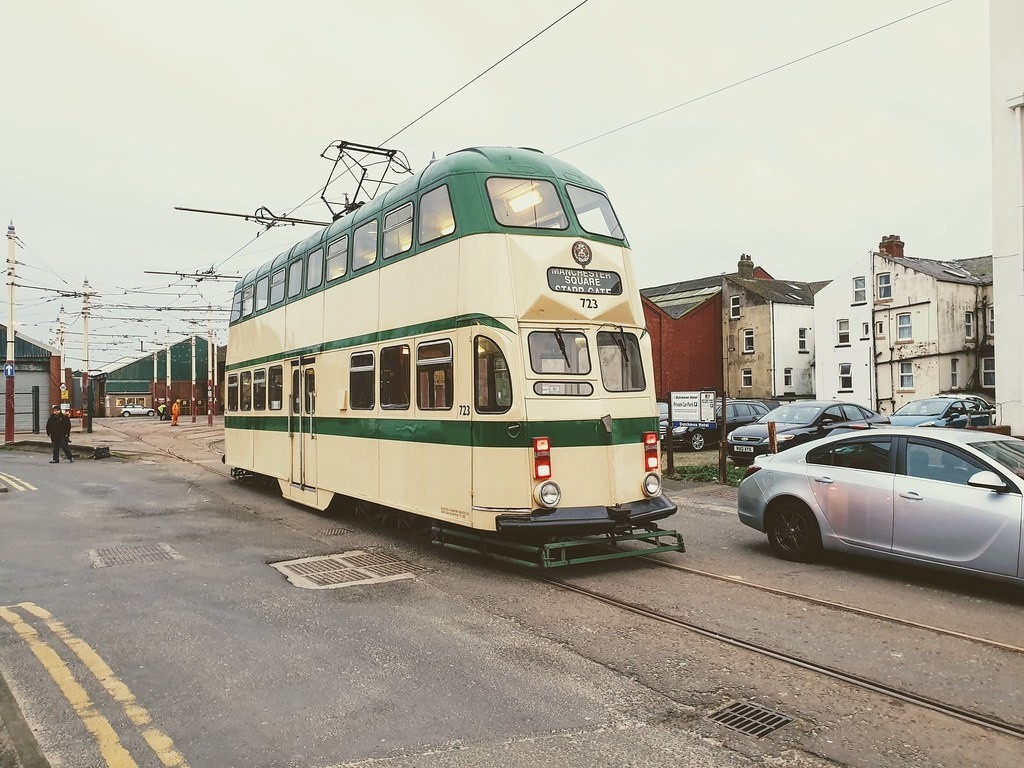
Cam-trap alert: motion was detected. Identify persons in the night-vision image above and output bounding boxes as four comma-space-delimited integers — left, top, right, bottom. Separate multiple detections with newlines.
158, 404, 167, 420
46, 406, 74, 463
170, 399, 181, 426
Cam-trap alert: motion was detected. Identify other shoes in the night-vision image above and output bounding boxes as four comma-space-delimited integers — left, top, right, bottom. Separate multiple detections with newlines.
49, 459, 59, 463
70, 457, 74, 463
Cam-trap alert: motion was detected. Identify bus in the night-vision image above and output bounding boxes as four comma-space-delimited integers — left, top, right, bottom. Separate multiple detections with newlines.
222, 138, 687, 570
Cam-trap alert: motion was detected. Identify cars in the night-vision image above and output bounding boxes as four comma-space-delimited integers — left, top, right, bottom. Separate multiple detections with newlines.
726, 400, 891, 467
659, 400, 771, 452
931, 393, 996, 426
736, 427, 1023, 589
887, 396, 991, 430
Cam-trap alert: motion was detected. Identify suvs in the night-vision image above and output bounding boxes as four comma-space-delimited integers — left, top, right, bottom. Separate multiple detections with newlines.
120, 403, 155, 417
